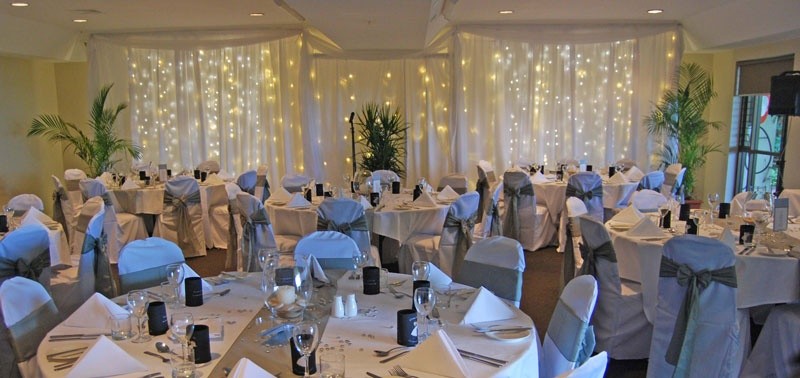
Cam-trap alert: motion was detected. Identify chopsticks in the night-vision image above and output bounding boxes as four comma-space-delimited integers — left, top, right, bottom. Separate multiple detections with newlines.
457, 348, 508, 368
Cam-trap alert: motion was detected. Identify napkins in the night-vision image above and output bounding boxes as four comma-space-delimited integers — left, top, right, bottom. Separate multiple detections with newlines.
624, 166, 644, 178
438, 185, 459, 199
398, 329, 470, 378
64, 292, 130, 328
303, 255, 329, 284
269, 188, 291, 199
67, 335, 148, 377
625, 216, 667, 236
176, 262, 212, 295
611, 204, 644, 223
608, 171, 628, 184
286, 191, 312, 207
417, 261, 453, 286
120, 177, 140, 190
455, 285, 517, 327
530, 172, 548, 184
356, 195, 373, 210
227, 357, 277, 378
412, 190, 437, 207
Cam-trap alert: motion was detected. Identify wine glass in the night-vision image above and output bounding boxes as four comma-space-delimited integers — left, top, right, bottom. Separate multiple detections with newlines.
615, 186, 800, 257
46, 248, 531, 378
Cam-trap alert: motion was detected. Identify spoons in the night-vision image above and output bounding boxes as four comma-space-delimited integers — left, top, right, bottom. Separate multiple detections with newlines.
202, 289, 231, 296
155, 341, 184, 359
373, 346, 409, 355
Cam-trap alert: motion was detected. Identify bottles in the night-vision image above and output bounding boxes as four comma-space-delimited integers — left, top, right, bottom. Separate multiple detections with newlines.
345, 294, 357, 318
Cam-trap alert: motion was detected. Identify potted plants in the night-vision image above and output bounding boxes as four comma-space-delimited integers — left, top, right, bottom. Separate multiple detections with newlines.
640, 61, 726, 209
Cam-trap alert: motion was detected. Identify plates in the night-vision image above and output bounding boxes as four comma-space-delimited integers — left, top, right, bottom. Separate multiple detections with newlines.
485, 325, 530, 343
218, 272, 251, 281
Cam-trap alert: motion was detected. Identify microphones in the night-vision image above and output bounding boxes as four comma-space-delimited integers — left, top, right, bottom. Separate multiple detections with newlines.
349, 112, 355, 122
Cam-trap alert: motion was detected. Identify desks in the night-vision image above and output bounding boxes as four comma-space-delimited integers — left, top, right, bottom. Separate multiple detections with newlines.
605, 210, 800, 326
39, 271, 539, 378
499, 172, 640, 245
264, 189, 451, 264
0, 209, 71, 277
110, 178, 230, 250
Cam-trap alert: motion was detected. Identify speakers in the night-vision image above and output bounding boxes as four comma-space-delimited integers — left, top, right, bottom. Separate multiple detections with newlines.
769, 75, 800, 116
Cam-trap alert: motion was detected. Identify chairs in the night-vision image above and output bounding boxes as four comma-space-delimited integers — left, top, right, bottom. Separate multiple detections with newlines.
0, 161, 800, 378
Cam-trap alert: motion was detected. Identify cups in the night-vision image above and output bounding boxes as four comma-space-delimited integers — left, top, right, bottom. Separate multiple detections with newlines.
0, 204, 16, 233
331, 295, 344, 318
609, 161, 625, 172
99, 163, 235, 190
272, 163, 460, 211
